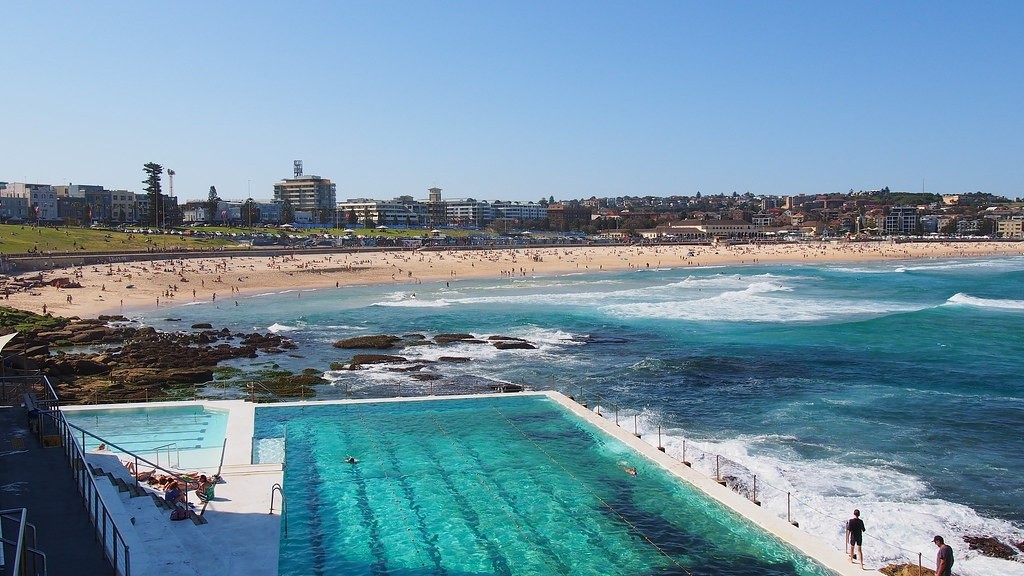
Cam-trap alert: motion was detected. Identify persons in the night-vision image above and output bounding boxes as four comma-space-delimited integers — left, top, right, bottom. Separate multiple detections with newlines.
94, 444, 105, 452
931, 535, 954, 576
0, 219, 1024, 314
847, 510, 866, 569
626, 467, 637, 475
124, 461, 214, 504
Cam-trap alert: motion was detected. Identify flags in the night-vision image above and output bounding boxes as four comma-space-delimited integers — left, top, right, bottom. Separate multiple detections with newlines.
35, 207, 39, 213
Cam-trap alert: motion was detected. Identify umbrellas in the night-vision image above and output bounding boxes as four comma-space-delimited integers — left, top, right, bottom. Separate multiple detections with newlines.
431, 230, 442, 233
521, 231, 532, 234
280, 224, 293, 228
377, 225, 388, 229
342, 229, 356, 233
